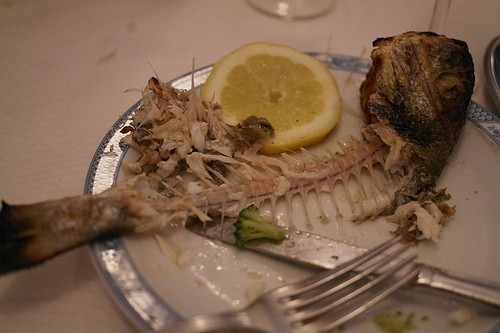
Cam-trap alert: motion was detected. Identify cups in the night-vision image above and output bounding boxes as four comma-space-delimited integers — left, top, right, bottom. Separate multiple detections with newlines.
248, 0, 336, 20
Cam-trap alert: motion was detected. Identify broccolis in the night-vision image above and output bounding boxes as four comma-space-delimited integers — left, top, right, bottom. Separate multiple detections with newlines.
236, 206, 286, 247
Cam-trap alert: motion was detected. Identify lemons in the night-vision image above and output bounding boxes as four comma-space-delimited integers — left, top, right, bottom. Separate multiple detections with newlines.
201, 42, 343, 152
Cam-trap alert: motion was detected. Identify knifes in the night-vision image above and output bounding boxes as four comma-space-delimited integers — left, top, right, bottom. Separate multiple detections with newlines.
184, 208, 499, 308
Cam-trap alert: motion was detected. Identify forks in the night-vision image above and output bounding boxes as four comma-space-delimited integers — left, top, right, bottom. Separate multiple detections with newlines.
155, 235, 420, 333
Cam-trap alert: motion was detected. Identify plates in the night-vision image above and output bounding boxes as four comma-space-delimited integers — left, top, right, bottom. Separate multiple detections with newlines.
83, 51, 500, 333
